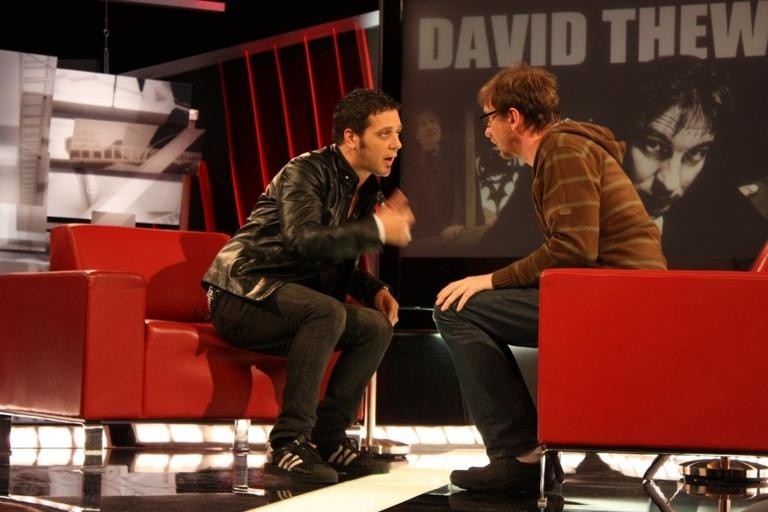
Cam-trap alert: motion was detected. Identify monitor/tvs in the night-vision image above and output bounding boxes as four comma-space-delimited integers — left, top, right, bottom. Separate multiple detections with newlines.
379, 0, 768, 329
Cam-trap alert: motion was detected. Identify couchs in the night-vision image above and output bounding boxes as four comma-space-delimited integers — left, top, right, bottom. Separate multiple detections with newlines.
1, 226, 385, 491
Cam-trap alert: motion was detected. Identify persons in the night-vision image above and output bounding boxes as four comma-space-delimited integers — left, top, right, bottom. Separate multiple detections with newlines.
399, 107, 485, 241
201, 87, 416, 483
480, 55, 768, 271
433, 62, 669, 491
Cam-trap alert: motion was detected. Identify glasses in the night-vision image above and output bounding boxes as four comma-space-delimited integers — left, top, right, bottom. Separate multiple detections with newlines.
480, 110, 496, 127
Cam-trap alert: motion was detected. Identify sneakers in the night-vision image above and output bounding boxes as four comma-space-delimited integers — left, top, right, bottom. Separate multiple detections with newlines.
311, 432, 389, 473
265, 434, 339, 484
450, 450, 564, 497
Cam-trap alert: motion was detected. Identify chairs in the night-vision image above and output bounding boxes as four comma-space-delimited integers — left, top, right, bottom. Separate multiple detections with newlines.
536, 235, 768, 509
